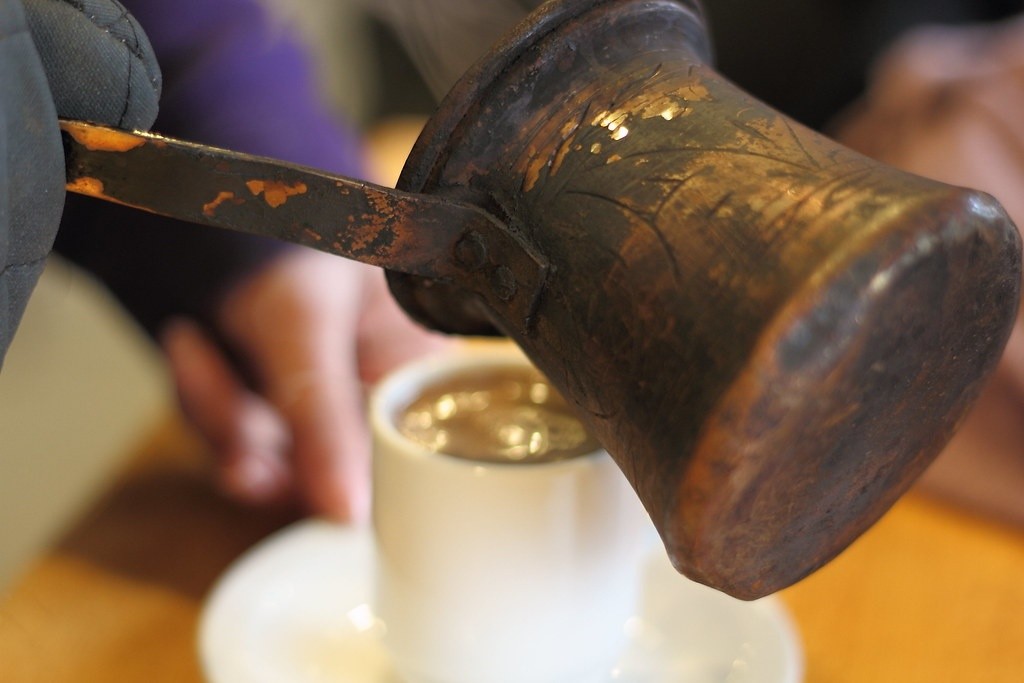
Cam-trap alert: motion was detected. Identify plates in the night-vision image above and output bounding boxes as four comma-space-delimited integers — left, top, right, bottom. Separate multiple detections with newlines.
196, 512, 798, 683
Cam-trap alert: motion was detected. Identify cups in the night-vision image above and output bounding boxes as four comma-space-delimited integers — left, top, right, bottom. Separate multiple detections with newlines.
364, 343, 649, 640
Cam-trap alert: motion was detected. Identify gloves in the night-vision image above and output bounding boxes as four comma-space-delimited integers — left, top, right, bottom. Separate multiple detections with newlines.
0, 1, 162, 370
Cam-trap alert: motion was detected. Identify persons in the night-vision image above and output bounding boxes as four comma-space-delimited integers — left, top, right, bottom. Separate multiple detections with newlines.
0, 1, 462, 533
